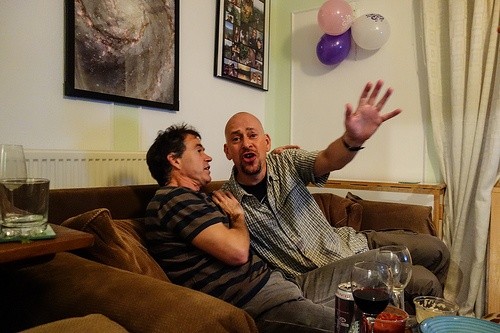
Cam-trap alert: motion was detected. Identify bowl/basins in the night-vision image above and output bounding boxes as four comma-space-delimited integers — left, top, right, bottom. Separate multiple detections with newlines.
413, 296, 460, 324
417, 315, 500, 333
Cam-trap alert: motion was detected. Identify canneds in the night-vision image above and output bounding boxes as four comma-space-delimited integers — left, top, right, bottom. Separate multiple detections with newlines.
334, 281, 366, 333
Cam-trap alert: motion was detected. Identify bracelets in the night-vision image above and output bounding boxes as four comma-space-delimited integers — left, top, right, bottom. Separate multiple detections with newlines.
341, 134, 365, 152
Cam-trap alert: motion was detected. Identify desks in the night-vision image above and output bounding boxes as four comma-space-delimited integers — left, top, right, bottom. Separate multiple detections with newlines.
0, 224, 92, 274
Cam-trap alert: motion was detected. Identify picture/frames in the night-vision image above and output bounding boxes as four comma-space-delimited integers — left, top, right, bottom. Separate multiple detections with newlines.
64, 0, 180, 110
213, 0, 271, 91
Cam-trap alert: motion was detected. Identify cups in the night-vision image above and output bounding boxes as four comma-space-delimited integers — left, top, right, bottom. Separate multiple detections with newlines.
359, 304, 409, 333
0, 177, 51, 243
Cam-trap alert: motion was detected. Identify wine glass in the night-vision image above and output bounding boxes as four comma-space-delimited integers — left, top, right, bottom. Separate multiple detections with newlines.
351, 261, 393, 333
373, 245, 412, 310
0, 144, 35, 214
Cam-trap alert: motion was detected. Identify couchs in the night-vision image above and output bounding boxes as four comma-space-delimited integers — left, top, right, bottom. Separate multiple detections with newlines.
21, 181, 437, 333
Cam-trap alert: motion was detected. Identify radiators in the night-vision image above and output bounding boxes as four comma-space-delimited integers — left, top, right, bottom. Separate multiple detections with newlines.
4, 151, 157, 191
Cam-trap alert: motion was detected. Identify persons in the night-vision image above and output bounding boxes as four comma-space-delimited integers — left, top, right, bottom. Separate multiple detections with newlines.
144, 125, 401, 333
213, 81, 449, 314
221, 0, 265, 84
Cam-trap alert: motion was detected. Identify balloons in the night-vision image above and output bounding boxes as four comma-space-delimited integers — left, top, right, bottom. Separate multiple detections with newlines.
317, 0, 353, 36
316, 27, 351, 65
351, 14, 389, 50
